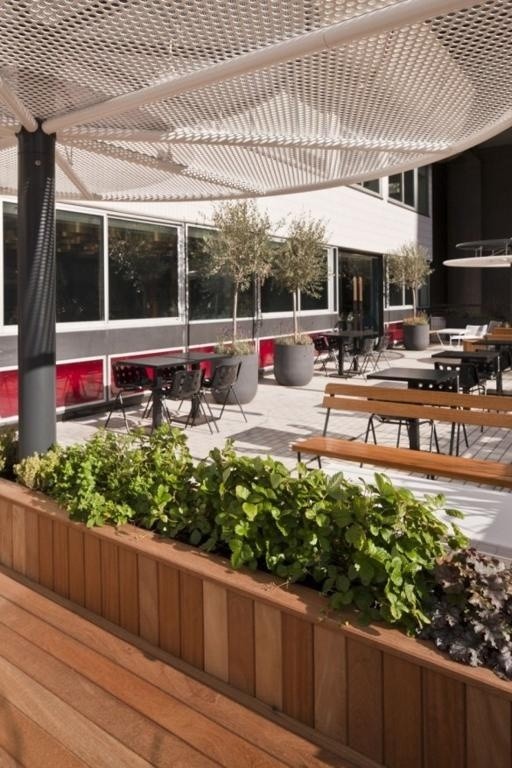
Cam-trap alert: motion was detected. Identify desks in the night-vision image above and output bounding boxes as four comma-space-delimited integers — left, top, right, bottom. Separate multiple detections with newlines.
117, 350, 230, 438
366, 338, 510, 448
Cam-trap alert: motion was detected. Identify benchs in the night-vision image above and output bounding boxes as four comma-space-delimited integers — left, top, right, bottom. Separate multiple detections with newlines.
292, 382, 511, 492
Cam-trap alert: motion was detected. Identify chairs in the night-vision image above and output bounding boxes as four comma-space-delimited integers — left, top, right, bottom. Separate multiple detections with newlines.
310, 321, 392, 380
104, 361, 248, 433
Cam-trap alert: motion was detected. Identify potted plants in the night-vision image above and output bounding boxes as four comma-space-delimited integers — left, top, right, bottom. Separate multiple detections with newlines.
383, 239, 435, 351
192, 192, 331, 405
